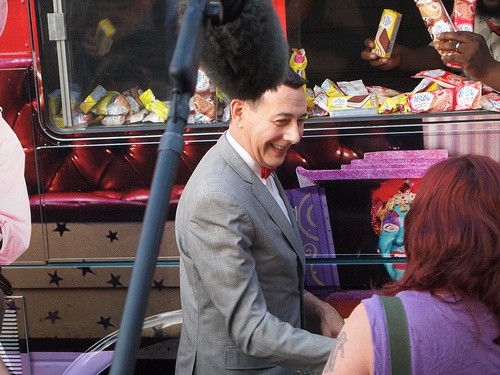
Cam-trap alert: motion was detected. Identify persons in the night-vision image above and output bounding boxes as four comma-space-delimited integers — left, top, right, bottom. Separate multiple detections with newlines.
325, 153, 500, 375
360, 0, 500, 92
173, 66, 345, 375
81, 0, 158, 92
371, 178, 420, 282
0, 108, 32, 375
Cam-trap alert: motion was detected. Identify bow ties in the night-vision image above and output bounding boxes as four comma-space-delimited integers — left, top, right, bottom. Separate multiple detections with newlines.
260, 166, 272, 178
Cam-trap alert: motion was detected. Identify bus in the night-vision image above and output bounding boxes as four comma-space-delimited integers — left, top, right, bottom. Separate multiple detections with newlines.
0, 0, 500, 362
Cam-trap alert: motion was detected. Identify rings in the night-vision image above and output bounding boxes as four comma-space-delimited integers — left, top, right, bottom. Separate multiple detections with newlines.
455, 42, 460, 52
